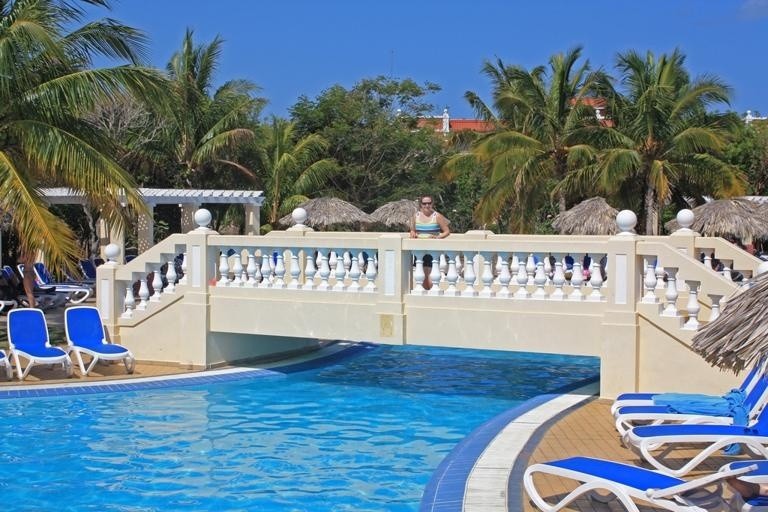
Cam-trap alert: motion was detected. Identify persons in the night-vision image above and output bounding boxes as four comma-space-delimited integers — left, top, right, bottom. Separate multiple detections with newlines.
15, 250, 38, 308
409, 195, 450, 290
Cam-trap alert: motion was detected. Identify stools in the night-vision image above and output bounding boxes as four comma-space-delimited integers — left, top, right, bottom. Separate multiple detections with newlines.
0, 349, 14, 380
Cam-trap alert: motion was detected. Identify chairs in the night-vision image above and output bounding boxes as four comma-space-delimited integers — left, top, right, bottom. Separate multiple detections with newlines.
520, 459, 767, 511
6, 308, 73, 382
0, 253, 138, 314
63, 306, 135, 376
610, 353, 768, 478
